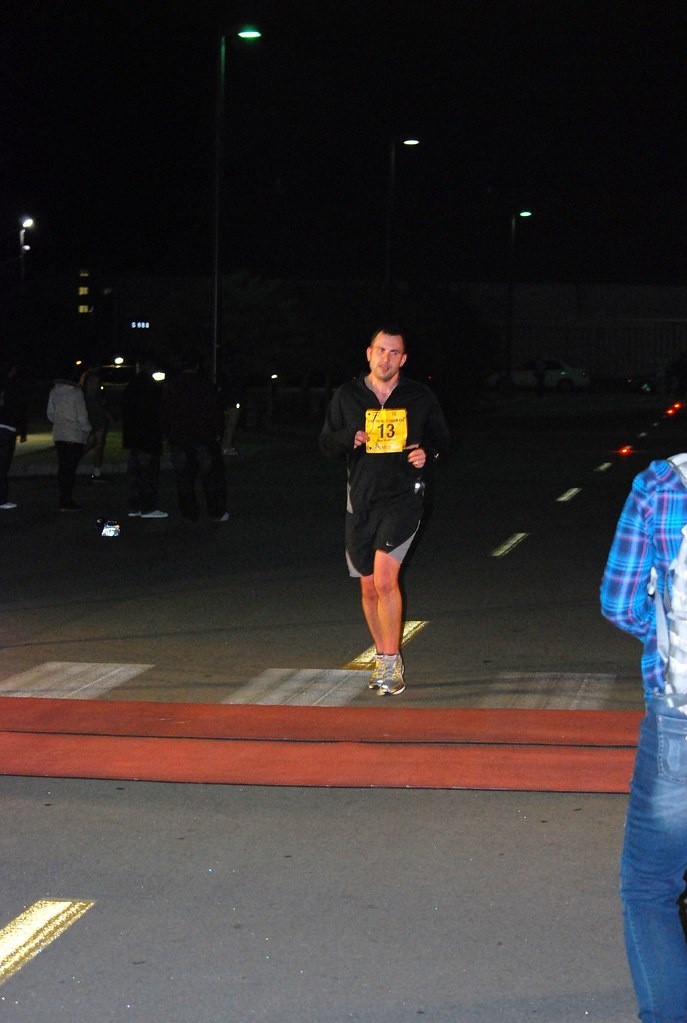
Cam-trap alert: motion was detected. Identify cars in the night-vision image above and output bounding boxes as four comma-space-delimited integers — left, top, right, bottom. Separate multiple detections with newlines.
504, 358, 596, 394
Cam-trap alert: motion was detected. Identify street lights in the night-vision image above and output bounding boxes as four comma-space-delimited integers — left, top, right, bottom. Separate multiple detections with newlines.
18, 216, 35, 254
211, 22, 263, 398
386, 136, 421, 328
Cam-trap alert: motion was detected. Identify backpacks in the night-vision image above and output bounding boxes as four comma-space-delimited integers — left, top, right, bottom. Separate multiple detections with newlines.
649, 452, 687, 724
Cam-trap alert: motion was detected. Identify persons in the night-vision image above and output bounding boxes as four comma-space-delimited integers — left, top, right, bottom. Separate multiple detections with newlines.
121, 361, 169, 518
0, 359, 27, 510
597, 452, 687, 1023
46, 367, 94, 512
158, 347, 230, 522
315, 329, 452, 698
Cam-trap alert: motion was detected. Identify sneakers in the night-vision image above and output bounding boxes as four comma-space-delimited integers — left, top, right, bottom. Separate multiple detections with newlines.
378, 654, 405, 695
369, 653, 384, 688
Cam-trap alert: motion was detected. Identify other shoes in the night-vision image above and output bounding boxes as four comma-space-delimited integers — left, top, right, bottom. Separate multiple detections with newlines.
141, 509, 169, 519
128, 510, 141, 517
0, 502, 18, 510
61, 503, 81, 512
214, 512, 229, 522
